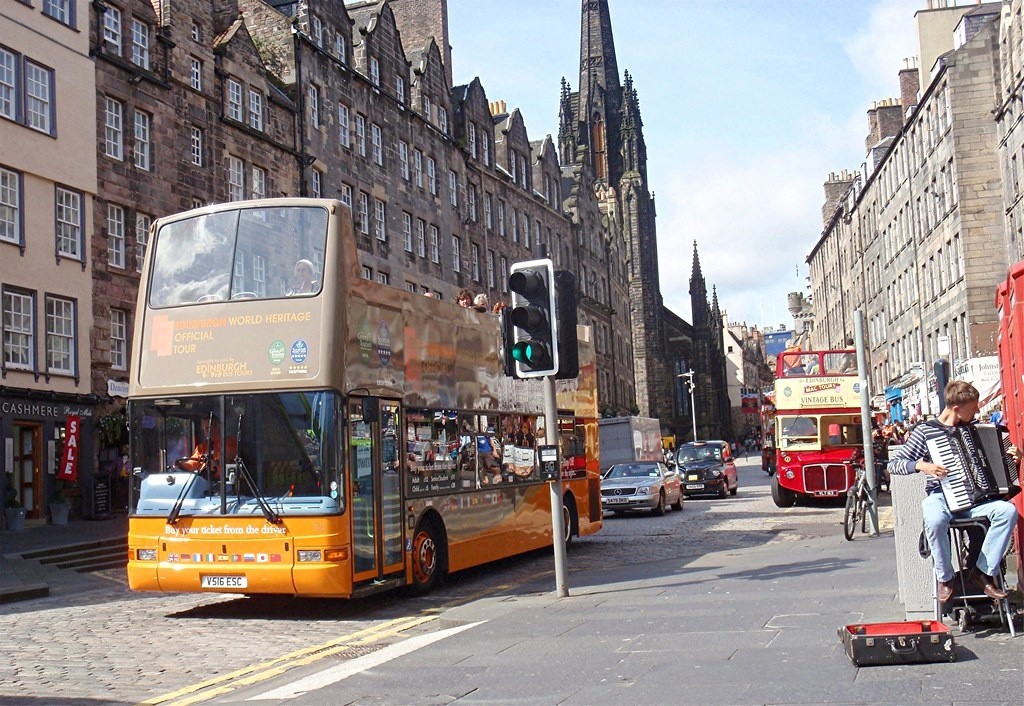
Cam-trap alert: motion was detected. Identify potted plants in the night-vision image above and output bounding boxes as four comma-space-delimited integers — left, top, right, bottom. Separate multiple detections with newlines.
5, 471, 27, 531
48, 481, 70, 525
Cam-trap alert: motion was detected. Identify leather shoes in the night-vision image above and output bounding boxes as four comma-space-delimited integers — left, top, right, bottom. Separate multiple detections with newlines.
968, 568, 1008, 599
938, 574, 958, 603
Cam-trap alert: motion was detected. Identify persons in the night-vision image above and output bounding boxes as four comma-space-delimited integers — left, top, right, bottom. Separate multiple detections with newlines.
172, 413, 239, 481
729, 432, 763, 455
662, 450, 677, 470
424, 289, 508, 315
106, 444, 130, 512
886, 380, 1024, 602
283, 259, 323, 296
783, 328, 858, 375
350, 397, 584, 490
869, 401, 1006, 494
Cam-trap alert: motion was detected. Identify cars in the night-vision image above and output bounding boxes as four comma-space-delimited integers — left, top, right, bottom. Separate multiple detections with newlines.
601, 461, 684, 515
288, 468, 320, 497
409, 439, 451, 475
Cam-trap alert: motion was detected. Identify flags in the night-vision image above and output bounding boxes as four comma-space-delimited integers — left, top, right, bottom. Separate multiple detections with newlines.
763, 390, 776, 417
741, 392, 759, 414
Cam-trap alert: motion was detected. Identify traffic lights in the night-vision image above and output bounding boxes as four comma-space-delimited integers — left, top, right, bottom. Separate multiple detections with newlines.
506, 263, 558, 378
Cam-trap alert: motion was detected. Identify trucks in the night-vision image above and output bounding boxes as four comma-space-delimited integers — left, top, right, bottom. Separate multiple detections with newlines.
599, 416, 665, 476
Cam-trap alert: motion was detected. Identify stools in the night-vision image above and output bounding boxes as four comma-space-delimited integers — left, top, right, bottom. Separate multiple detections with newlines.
919, 517, 1015, 637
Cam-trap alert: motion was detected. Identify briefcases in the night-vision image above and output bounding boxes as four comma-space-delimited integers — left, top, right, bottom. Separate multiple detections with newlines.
837, 621, 955, 667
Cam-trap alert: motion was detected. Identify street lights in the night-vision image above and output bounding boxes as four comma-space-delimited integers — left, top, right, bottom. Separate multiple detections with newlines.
678, 370, 697, 442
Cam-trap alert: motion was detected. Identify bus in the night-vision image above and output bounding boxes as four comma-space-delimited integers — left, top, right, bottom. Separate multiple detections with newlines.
126, 202, 602, 600
770, 349, 866, 507
761, 399, 777, 473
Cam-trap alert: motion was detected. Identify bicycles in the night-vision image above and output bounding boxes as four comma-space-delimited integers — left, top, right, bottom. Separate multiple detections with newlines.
841, 444, 879, 541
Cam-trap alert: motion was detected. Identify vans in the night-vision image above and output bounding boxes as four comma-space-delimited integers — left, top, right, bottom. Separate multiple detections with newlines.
674, 441, 739, 499
